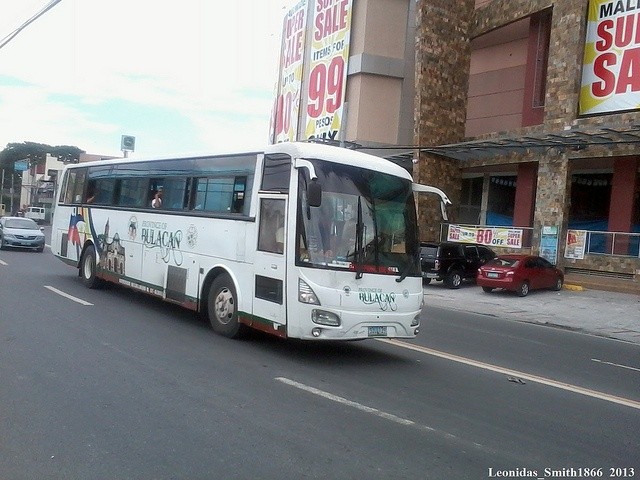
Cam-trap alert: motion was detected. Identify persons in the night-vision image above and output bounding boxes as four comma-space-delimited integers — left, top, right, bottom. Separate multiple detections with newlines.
87, 190, 99, 203
151, 190, 163, 209
275, 226, 333, 259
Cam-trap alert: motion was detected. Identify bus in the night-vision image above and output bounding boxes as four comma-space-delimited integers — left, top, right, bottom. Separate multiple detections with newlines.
50, 142, 452, 342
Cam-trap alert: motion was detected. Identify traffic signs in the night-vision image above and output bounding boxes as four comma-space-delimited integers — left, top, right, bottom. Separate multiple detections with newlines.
14, 162, 28, 171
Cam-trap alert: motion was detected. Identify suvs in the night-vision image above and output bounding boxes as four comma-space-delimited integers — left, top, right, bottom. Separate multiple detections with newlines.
25, 207, 45, 222
420, 241, 499, 290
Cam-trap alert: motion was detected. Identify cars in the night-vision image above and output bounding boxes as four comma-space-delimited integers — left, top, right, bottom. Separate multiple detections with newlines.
0, 216, 46, 253
476, 254, 565, 297
